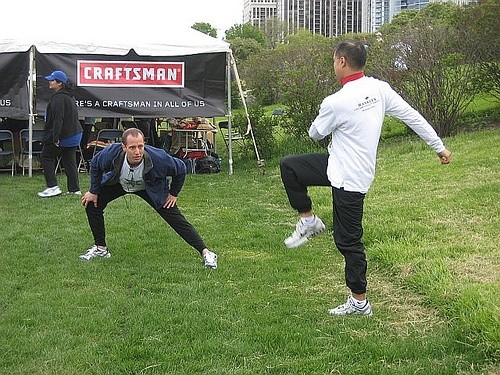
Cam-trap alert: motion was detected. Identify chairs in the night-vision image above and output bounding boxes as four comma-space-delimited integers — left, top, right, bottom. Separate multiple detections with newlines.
270, 108, 284, 126
219, 119, 246, 155
0, 120, 155, 178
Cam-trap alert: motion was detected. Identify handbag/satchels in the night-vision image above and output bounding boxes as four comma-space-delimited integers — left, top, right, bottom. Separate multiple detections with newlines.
195, 159, 220, 174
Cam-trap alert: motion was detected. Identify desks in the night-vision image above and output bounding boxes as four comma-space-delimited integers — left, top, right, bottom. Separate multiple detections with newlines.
173, 129, 210, 159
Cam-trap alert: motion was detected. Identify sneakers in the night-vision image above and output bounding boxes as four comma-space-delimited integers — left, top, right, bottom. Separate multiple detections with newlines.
284, 214, 325, 248
203, 250, 218, 270
79, 244, 111, 260
328, 295, 373, 316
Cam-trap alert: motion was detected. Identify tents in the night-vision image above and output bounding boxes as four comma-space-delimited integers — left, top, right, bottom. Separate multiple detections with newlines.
0, 0, 233, 175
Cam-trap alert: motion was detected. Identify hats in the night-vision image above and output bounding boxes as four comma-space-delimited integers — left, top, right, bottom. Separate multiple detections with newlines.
45, 71, 68, 84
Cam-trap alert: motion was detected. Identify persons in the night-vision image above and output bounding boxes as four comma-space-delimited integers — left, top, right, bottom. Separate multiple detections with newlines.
37, 71, 83, 197
280, 41, 452, 316
79, 128, 217, 269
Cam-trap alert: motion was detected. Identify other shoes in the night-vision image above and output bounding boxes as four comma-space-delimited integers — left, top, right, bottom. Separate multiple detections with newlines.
62, 190, 82, 195
37, 185, 62, 197
